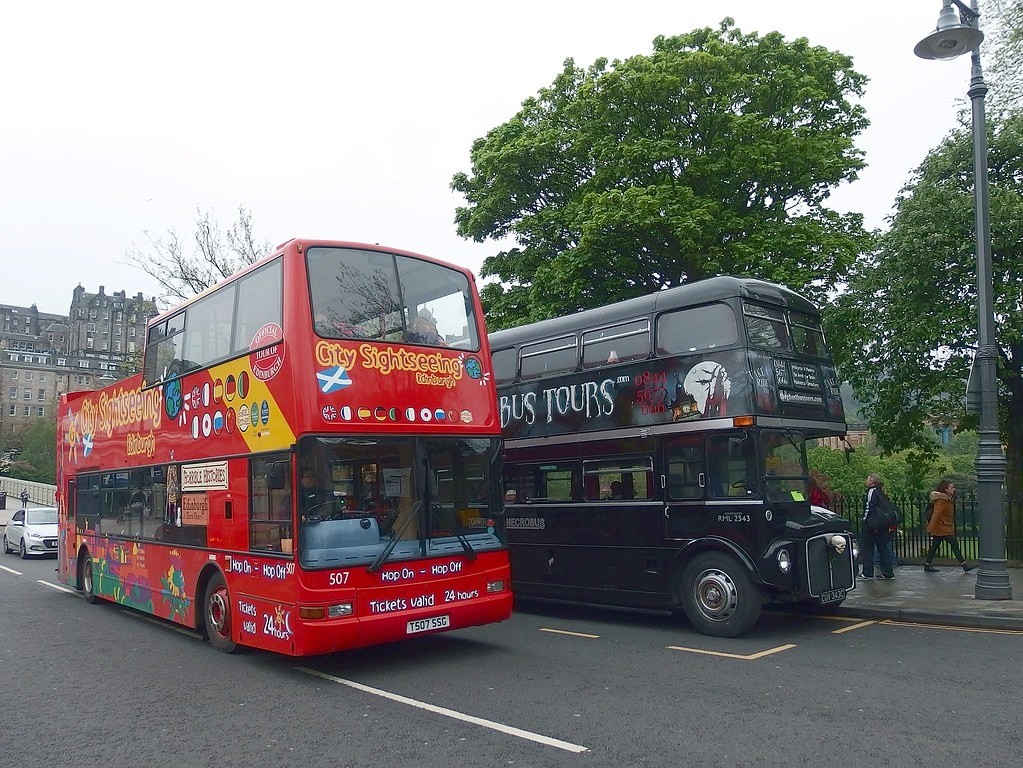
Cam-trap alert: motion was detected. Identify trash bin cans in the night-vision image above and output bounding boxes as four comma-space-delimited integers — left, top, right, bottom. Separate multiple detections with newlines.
0, 491, 6, 510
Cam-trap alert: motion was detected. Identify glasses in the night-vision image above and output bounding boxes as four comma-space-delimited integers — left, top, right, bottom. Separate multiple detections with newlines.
303, 474, 315, 479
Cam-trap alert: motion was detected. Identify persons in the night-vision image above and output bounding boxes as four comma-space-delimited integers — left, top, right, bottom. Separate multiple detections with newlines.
922, 479, 976, 573
809, 469, 831, 506
856, 474, 898, 581
299, 465, 349, 523
610, 481, 624, 496
21, 488, 30, 509
505, 489, 516, 502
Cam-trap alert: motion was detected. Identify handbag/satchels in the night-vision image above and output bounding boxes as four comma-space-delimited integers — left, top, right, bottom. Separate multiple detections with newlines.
865, 487, 904, 536
820, 491, 831, 505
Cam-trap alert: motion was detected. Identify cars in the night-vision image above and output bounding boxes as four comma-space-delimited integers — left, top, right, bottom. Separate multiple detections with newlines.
3, 508, 58, 560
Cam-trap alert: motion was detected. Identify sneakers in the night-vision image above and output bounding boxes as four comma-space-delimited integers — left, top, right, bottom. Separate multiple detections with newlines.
857, 573, 873, 579
875, 574, 895, 579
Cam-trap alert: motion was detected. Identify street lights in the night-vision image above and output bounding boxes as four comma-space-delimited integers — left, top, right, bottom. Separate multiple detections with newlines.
914, 0, 1014, 600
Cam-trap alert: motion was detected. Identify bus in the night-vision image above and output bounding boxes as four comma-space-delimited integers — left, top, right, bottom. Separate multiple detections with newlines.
431, 276, 860, 639
55, 236, 513, 659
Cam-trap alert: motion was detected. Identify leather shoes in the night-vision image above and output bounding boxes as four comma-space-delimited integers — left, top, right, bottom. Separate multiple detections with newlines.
962, 563, 975, 572
924, 563, 939, 572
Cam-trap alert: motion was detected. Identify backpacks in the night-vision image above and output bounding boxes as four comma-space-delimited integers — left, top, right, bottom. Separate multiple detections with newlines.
924, 502, 934, 523
